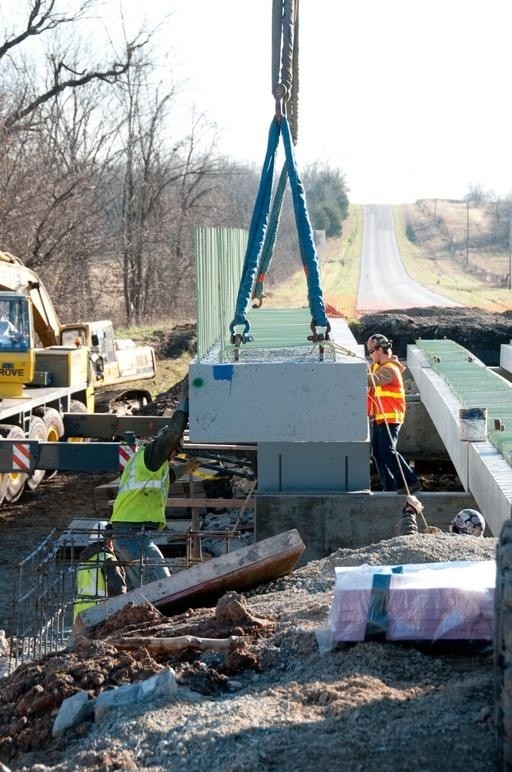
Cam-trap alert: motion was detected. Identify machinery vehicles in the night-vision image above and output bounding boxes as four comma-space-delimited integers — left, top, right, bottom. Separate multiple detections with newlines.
0, 250, 156, 506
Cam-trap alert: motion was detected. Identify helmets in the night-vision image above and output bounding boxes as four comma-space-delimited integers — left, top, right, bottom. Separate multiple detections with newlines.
367, 333, 393, 351
88, 520, 111, 542
148, 426, 185, 451
451, 508, 486, 539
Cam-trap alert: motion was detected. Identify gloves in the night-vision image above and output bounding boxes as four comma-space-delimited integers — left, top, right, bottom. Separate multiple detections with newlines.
180, 373, 190, 400
403, 494, 424, 515
422, 525, 441, 534
184, 456, 202, 474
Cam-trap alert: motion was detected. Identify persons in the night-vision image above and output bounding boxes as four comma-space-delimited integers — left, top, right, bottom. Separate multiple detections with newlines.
108, 374, 189, 593
0, 314, 20, 338
397, 496, 486, 539
367, 334, 424, 492
73, 539, 127, 627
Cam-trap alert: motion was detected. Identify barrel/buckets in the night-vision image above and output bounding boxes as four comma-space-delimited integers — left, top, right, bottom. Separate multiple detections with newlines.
459, 408, 487, 441
